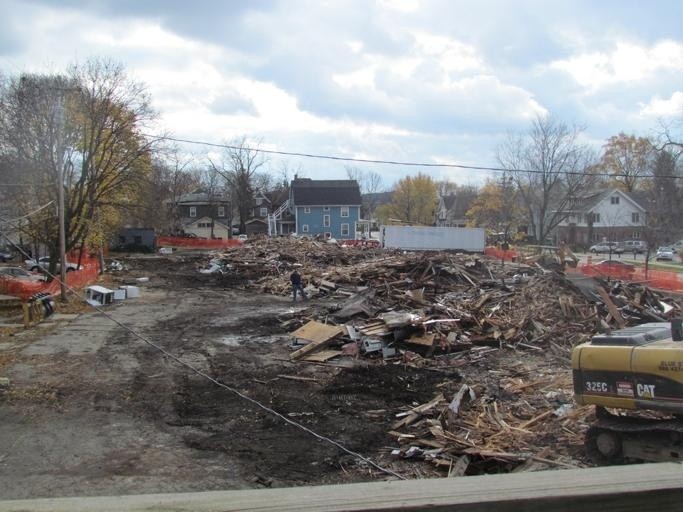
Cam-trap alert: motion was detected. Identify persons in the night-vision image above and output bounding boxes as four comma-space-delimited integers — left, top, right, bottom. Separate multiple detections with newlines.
289, 271, 308, 302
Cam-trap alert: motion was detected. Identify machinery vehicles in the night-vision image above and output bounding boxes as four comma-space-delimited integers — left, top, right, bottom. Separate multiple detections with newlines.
567, 316, 683, 467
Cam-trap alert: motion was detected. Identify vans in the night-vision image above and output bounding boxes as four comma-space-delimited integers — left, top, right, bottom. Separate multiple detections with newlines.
587, 239, 683, 261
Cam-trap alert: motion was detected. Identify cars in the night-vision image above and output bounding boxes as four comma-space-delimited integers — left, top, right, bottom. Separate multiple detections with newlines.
0, 266, 48, 282
24, 254, 84, 274
232, 227, 240, 235
238, 234, 248, 241
0, 250, 15, 263
